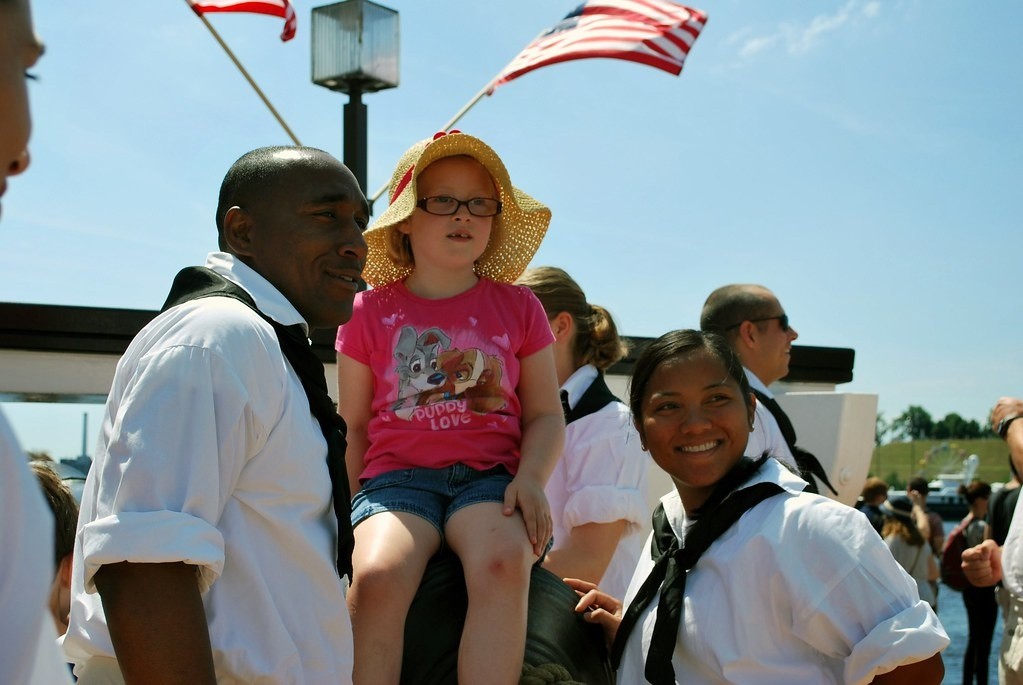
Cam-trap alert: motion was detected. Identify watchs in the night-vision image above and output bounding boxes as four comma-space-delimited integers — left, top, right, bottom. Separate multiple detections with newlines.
996, 413, 1023, 439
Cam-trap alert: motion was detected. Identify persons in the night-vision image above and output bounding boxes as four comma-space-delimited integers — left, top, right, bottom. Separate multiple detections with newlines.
0, 0, 79, 685
332, 129, 564, 685
517, 266, 655, 607
562, 327, 952, 685
700, 283, 839, 499
857, 396, 1023, 685
56, 143, 371, 685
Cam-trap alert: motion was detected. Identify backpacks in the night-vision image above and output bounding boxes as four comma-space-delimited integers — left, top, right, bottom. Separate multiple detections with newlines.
940, 517, 980, 592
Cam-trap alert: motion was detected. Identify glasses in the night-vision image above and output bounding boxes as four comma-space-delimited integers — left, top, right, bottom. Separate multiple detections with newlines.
725, 314, 788, 333
415, 194, 501, 217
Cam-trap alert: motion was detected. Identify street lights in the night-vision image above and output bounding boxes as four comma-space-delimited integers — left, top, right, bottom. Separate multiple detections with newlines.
311, 0, 401, 194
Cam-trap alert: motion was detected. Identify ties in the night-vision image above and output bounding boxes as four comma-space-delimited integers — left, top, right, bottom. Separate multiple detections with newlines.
561, 368, 623, 424
604, 482, 782, 685
751, 388, 839, 497
163, 266, 355, 587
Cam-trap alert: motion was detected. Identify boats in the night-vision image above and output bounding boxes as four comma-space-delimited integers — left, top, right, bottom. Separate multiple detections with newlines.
884, 454, 980, 522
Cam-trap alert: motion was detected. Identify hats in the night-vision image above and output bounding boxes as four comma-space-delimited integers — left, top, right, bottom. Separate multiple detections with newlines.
878, 496, 914, 525
360, 130, 552, 285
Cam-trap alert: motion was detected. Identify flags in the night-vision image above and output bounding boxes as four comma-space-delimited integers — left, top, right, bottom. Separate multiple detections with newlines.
187, 0, 296, 42
487, 0, 708, 86
